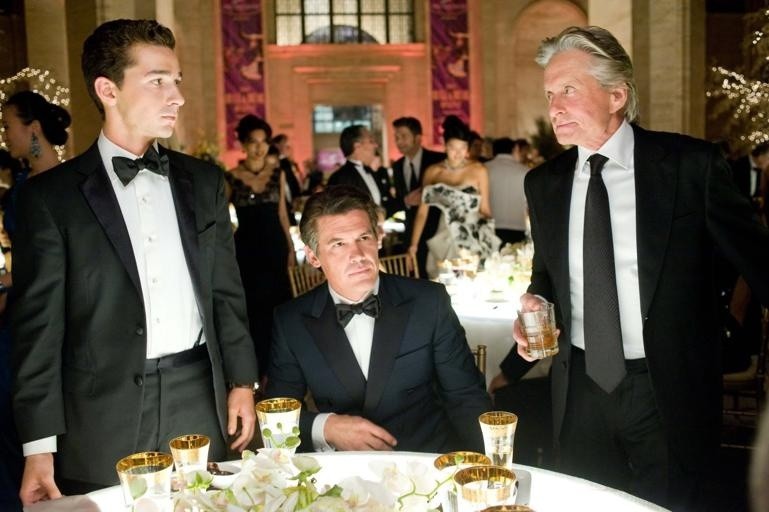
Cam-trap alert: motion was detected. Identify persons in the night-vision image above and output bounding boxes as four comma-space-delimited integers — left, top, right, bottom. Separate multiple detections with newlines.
467, 130, 489, 163
219, 112, 296, 386
301, 161, 323, 195
388, 116, 446, 279
483, 137, 531, 251
735, 144, 769, 199
264, 187, 494, 453
486, 340, 555, 463
0, 87, 75, 312
512, 24, 769, 512
325, 124, 393, 258
405, 115, 490, 274
0, 16, 259, 506
515, 139, 536, 170
268, 134, 300, 200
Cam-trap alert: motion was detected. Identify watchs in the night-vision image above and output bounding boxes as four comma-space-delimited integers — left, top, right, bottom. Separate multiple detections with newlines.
227, 381, 259, 390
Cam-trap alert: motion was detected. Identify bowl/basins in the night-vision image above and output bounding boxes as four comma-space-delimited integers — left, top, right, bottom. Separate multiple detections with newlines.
208, 463, 241, 489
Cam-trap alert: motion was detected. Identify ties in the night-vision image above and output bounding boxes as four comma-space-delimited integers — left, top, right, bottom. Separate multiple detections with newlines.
408, 161, 418, 213
583, 153, 627, 394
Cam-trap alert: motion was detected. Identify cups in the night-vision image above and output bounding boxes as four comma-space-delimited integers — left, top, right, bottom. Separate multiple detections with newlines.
515, 301, 560, 360
435, 450, 490, 492
455, 464, 518, 512
170, 434, 211, 474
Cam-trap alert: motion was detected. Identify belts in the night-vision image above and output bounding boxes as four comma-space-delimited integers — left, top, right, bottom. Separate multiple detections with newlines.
146, 342, 208, 375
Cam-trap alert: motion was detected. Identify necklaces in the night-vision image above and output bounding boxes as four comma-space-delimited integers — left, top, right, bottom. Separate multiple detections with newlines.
444, 159, 465, 175
244, 160, 267, 176
26, 160, 62, 177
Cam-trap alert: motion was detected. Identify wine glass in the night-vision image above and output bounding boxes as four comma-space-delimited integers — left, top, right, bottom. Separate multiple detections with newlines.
479, 413, 518, 463
254, 396, 302, 454
115, 451, 174, 499
481, 504, 533, 512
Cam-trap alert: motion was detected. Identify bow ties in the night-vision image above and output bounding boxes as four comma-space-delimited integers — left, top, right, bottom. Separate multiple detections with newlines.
112, 143, 170, 187
363, 165, 373, 174
753, 167, 761, 172
335, 294, 383, 328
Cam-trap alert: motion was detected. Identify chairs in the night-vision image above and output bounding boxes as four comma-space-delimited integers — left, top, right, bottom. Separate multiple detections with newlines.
286, 264, 327, 300
377, 252, 421, 279
